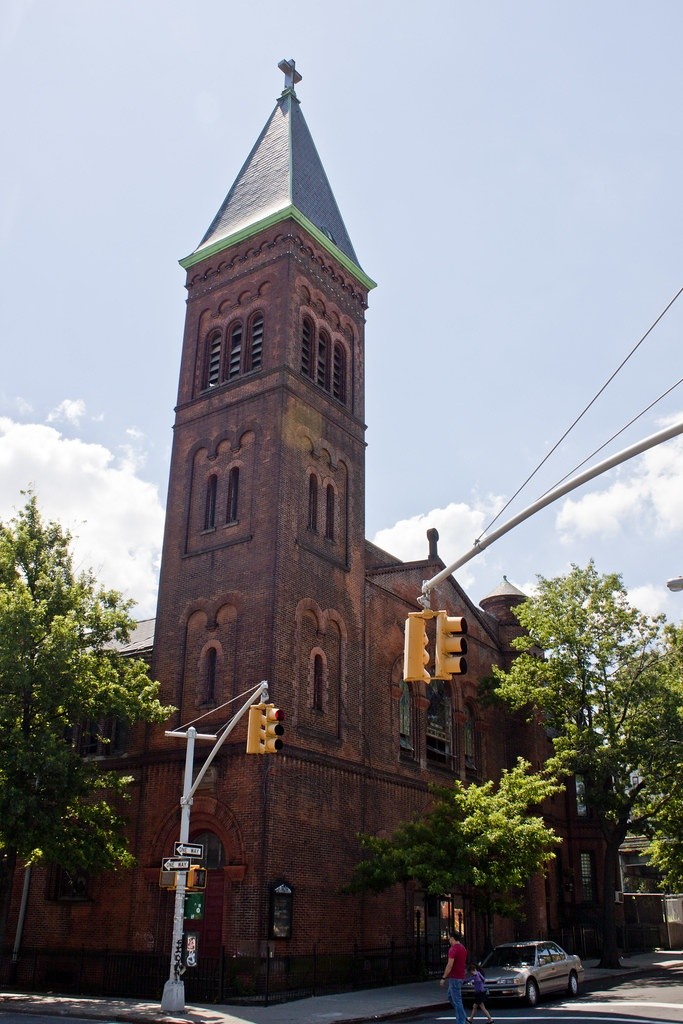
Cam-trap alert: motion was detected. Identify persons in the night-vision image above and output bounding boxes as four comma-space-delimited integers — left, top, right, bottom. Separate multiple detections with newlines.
463, 962, 495, 1024
439, 932, 467, 1024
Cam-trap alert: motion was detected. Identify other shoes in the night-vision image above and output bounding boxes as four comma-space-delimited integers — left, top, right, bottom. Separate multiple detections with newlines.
465, 1017, 471, 1024
485, 1021, 495, 1024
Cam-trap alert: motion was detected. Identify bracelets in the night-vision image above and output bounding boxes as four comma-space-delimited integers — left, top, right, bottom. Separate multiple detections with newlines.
440, 976, 446, 980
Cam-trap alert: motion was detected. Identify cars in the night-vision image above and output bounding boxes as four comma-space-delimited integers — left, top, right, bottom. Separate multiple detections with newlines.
460, 940, 587, 1009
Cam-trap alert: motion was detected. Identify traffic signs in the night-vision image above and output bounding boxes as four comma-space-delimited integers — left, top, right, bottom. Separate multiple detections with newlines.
160, 841, 204, 873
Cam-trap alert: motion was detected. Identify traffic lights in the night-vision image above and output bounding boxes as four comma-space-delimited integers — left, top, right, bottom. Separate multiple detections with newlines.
247, 704, 284, 756
405, 609, 469, 687
